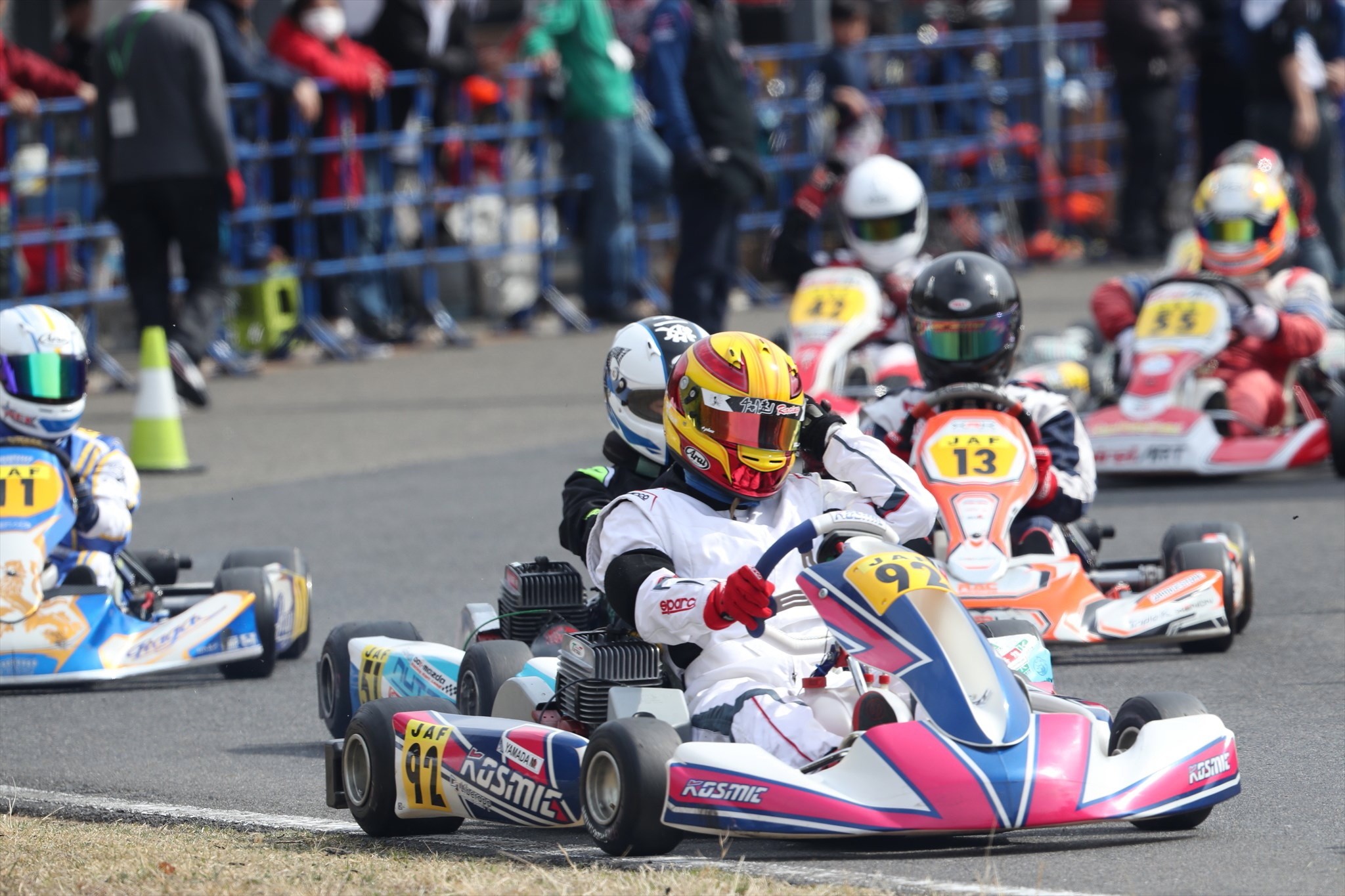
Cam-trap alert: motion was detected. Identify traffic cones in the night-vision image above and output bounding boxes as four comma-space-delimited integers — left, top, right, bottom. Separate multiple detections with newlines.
125, 324, 203, 474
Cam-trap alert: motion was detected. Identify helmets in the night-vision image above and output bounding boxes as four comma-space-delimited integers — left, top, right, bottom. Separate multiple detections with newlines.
842, 153, 929, 273
909, 251, 1022, 390
603, 315, 712, 467
664, 331, 807, 502
0, 305, 89, 437
1216, 140, 1302, 209
1192, 164, 1297, 273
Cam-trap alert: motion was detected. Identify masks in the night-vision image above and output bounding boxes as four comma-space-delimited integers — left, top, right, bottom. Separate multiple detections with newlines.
299, 6, 346, 43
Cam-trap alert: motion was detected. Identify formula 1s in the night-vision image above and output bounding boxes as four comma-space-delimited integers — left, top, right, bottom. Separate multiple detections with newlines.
881, 380, 1261, 667
0, 434, 319, 685
1056, 274, 1332, 480
742, 247, 928, 428
316, 503, 1259, 857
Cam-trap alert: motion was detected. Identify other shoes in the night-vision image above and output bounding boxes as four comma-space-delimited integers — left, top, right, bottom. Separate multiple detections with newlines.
161, 339, 208, 407
901, 535, 937, 559
1015, 526, 1055, 557
852, 690, 899, 732
61, 564, 97, 587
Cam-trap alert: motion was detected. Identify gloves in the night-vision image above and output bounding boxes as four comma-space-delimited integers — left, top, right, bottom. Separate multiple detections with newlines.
1236, 302, 1282, 342
801, 393, 846, 460
1032, 444, 1059, 506
715, 566, 776, 631
74, 476, 99, 530
1113, 326, 1150, 378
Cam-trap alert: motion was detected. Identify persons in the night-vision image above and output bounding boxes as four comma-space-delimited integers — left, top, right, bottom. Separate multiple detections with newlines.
0, 0, 1345, 413
1090, 165, 1332, 436
560, 314, 709, 558
859, 252, 1097, 567
0, 305, 140, 609
585, 331, 1035, 769
